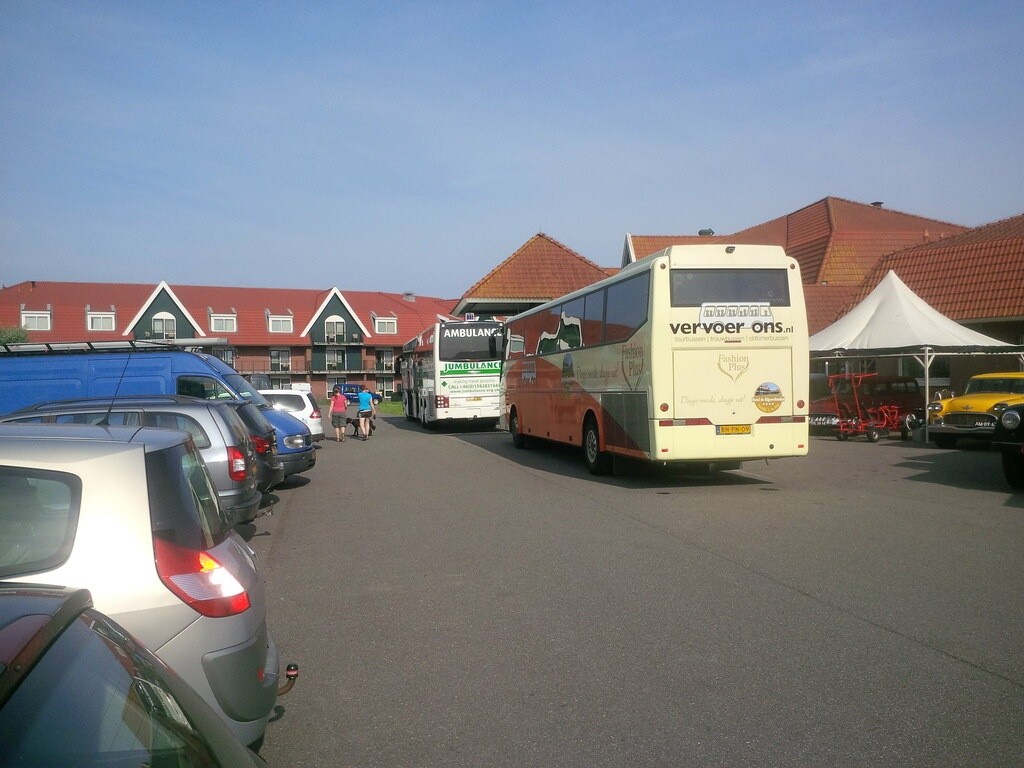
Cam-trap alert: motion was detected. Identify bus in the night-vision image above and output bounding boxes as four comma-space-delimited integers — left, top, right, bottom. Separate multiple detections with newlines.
488, 246, 810, 473
395, 320, 504, 428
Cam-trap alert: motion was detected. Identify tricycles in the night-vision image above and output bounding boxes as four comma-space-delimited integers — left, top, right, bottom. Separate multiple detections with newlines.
833, 373, 908, 441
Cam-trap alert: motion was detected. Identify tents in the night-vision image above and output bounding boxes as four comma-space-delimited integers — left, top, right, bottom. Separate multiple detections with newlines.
808, 271, 1024, 443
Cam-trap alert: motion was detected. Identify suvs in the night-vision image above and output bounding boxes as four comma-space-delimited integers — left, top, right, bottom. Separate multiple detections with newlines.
1, 394, 262, 527
0, 423, 299, 756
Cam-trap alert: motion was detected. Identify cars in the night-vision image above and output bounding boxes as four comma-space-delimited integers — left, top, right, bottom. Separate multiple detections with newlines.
207, 390, 326, 442
999, 402, 1024, 481
930, 373, 1024, 444
808, 374, 925, 437
212, 398, 284, 496
336, 383, 382, 405
1, 582, 273, 768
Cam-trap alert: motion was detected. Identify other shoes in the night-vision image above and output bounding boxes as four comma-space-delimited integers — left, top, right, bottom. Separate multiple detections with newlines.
342, 433, 345, 442
336, 438, 341, 442
361, 435, 368, 441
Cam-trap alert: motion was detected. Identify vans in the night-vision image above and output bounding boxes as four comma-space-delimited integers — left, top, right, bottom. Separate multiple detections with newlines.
1, 338, 316, 482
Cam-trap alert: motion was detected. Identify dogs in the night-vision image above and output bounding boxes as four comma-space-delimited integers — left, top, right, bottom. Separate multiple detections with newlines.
346, 417, 376, 438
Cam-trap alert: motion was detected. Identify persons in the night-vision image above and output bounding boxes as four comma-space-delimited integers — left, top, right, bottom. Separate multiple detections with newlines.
358, 385, 376, 441
328, 388, 348, 442
